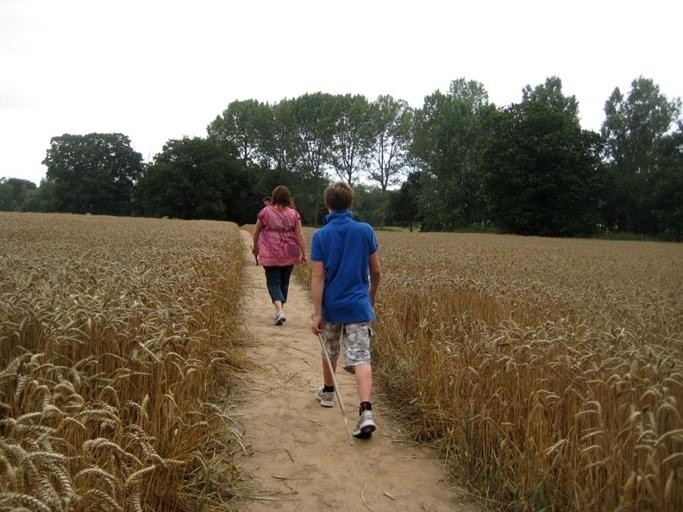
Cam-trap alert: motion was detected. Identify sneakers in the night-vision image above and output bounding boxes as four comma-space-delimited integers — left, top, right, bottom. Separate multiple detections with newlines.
317, 384, 336, 407
352, 410, 376, 438
273, 312, 286, 325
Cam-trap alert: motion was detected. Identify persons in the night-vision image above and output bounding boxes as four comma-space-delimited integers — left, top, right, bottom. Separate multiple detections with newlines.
258, 195, 272, 207
307, 179, 383, 441
250, 185, 307, 326
